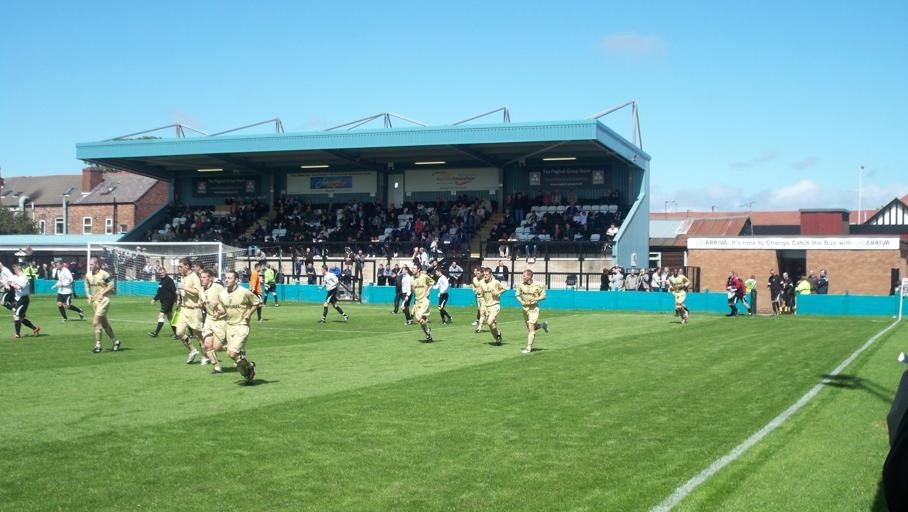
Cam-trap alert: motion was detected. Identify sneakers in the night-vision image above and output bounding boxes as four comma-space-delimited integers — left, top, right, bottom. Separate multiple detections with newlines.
170, 333, 180, 340
33, 325, 40, 337
317, 320, 326, 324
201, 353, 257, 383
472, 319, 501, 345
520, 348, 533, 355
342, 313, 349, 322
91, 346, 103, 354
112, 340, 121, 352
15, 334, 21, 338
542, 321, 551, 336
186, 348, 200, 364
390, 308, 453, 344
148, 331, 158, 337
80, 308, 84, 320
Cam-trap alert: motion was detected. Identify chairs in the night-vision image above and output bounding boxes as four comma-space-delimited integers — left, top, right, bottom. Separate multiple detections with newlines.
144, 198, 622, 257
